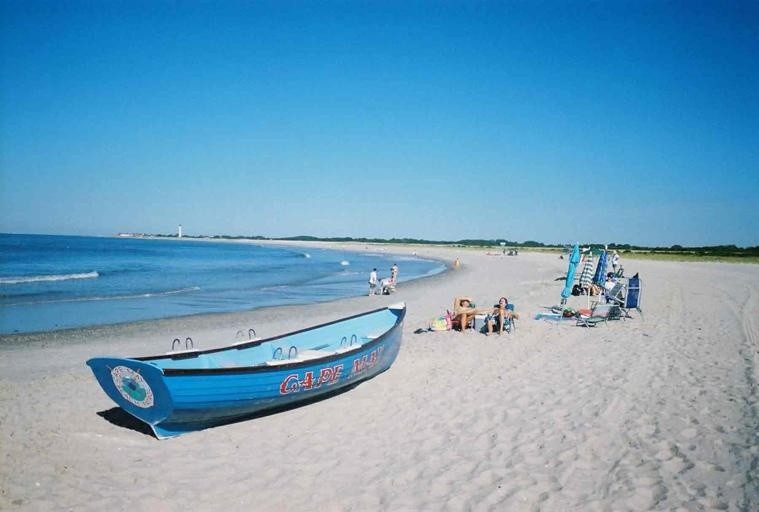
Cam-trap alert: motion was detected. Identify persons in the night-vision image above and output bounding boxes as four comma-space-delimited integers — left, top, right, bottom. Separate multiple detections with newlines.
446, 295, 479, 332
484, 296, 518, 337
392, 263, 399, 280
388, 275, 395, 289
590, 281, 602, 296
454, 257, 461, 273
611, 249, 620, 274
377, 278, 392, 296
369, 267, 377, 297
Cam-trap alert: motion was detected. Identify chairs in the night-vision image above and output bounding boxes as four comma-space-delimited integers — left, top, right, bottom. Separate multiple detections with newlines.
466, 300, 517, 336
573, 303, 614, 330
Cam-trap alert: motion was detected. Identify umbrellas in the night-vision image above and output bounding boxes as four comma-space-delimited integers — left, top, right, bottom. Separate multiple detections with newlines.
592, 243, 610, 303
556, 241, 581, 319
578, 246, 594, 310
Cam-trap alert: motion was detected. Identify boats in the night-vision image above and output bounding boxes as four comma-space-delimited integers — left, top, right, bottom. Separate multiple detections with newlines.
85, 300, 406, 440
